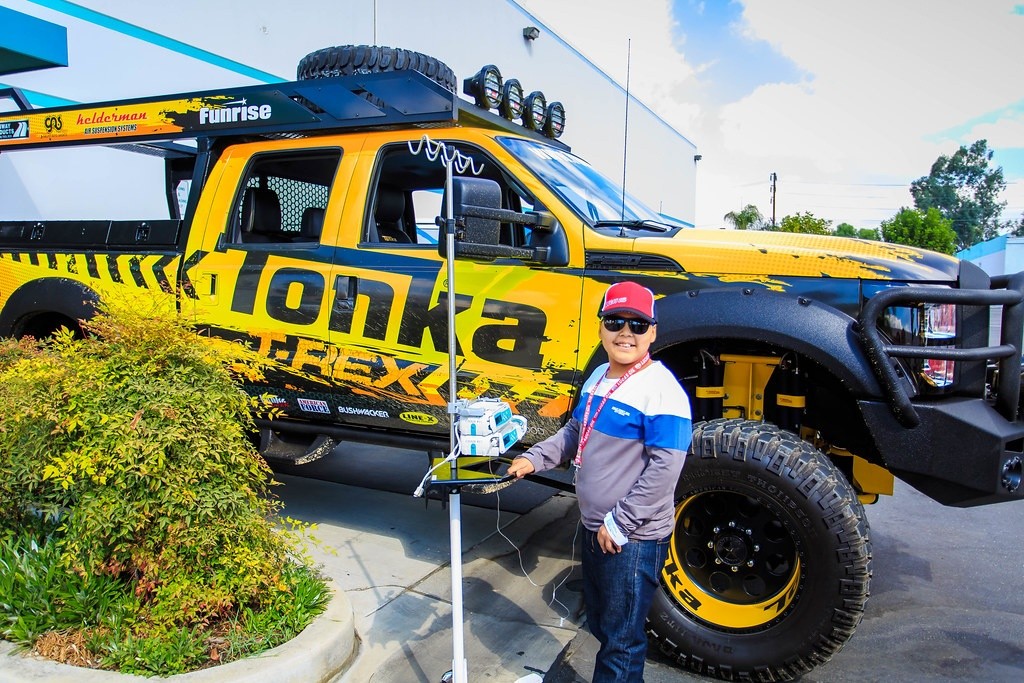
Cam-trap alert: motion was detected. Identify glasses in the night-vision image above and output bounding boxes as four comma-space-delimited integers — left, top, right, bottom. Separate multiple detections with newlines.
604, 314, 650, 334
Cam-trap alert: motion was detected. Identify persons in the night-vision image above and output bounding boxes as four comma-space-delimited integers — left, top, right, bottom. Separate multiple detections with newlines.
507, 281, 692, 683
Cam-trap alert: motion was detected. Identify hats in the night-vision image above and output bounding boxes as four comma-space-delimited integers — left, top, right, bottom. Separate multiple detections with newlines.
597, 281, 658, 324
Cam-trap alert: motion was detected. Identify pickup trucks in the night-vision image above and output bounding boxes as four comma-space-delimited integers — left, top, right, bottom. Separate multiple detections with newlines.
1, 43, 1024, 683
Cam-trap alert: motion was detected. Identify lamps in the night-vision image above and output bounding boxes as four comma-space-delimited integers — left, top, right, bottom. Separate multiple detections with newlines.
694, 155, 702, 161
523, 27, 540, 40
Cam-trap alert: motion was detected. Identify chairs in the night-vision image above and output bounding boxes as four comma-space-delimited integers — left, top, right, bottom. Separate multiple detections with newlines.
374, 183, 412, 243
240, 187, 293, 243
293, 208, 325, 242
